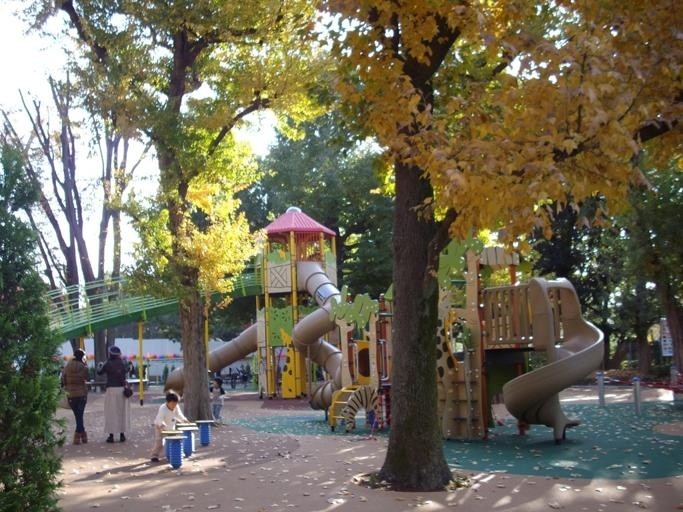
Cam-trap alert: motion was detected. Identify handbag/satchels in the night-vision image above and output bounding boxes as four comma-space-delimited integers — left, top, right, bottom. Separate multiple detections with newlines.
123, 388, 133, 397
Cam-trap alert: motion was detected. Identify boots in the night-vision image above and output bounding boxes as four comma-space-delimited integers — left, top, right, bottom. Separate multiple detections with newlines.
80, 431, 87, 444
73, 431, 82, 445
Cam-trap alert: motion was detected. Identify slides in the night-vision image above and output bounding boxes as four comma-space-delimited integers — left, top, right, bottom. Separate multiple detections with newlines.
502, 322, 605, 440
164, 322, 257, 397
293, 261, 343, 410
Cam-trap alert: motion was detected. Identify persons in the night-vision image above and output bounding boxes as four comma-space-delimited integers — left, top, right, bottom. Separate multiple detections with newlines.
59, 347, 89, 446
95, 360, 106, 393
209, 376, 227, 423
59, 366, 65, 388
95, 346, 133, 443
126, 360, 135, 379
150, 388, 190, 463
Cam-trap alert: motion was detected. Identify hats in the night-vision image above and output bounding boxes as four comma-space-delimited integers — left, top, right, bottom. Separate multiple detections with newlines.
74, 349, 85, 357
109, 347, 121, 355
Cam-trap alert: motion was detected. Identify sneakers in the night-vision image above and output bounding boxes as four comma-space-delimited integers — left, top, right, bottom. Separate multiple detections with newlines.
106, 436, 114, 442
119, 435, 126, 442
